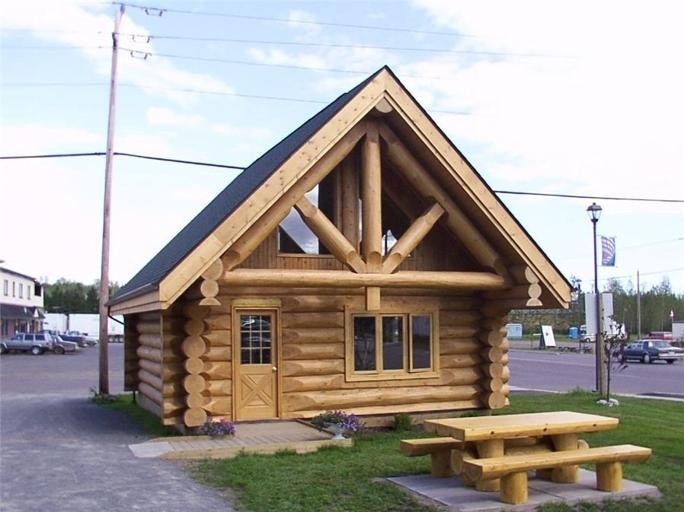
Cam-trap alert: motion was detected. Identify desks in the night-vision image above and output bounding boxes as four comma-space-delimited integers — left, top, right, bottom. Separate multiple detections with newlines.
423, 411, 619, 492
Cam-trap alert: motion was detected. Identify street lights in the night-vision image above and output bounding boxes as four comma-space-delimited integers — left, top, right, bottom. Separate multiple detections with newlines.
585, 201, 603, 393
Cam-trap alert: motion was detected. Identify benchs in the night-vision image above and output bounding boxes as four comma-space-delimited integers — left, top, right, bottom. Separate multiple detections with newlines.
401, 436, 537, 476
464, 443, 652, 504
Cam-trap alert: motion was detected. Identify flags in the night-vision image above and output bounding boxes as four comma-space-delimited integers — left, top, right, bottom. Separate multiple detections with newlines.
601, 236, 616, 266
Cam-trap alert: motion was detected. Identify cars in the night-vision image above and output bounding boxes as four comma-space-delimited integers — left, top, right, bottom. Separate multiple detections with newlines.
0, 331, 99, 355
240, 317, 270, 362
612, 340, 684, 363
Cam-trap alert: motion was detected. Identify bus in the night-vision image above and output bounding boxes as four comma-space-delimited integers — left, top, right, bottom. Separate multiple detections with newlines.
581, 324, 625, 341
